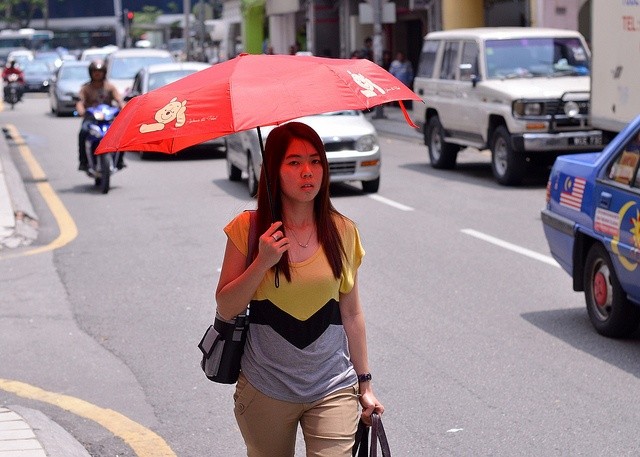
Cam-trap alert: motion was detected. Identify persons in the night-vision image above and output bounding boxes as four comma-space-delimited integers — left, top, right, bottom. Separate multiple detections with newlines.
77, 61, 125, 171
1, 60, 24, 81
214, 122, 383, 456
390, 53, 413, 91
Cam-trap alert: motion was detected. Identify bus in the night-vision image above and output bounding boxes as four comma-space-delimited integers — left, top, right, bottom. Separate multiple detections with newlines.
0, 28, 54, 67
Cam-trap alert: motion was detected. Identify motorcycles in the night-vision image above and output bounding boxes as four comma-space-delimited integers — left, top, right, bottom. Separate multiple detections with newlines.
3, 72, 25, 109
73, 103, 128, 193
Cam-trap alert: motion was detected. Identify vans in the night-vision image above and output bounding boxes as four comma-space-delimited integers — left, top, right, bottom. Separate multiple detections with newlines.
6, 49, 32, 69
102, 47, 170, 96
78, 47, 119, 64
411, 26, 603, 188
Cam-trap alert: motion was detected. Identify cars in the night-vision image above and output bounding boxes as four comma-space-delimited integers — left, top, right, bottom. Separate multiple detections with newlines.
226, 108, 381, 195
19, 61, 50, 90
122, 60, 226, 159
34, 51, 61, 72
47, 60, 92, 117
539, 114, 639, 338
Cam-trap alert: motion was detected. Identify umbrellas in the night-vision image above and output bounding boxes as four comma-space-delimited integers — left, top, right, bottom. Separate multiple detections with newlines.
94, 55, 426, 152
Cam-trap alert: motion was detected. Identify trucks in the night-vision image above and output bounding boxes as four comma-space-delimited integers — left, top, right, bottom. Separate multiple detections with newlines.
590, 0, 639, 144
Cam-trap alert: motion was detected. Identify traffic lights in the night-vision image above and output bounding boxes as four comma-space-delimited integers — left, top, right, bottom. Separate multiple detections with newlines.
128, 11, 133, 22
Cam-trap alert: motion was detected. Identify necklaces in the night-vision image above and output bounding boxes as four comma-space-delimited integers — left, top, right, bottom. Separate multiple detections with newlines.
284, 224, 317, 249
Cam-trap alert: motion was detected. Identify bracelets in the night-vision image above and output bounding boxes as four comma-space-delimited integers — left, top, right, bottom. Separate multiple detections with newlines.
356, 373, 373, 381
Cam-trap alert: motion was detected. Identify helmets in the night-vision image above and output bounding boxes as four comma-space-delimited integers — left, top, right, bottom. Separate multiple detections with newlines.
88, 59, 107, 80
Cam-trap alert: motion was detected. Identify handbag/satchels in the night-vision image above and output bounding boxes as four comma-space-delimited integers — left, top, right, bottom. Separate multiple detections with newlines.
198, 303, 250, 383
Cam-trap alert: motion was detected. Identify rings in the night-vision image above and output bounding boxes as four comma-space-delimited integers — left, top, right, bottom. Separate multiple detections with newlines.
273, 233, 281, 242
